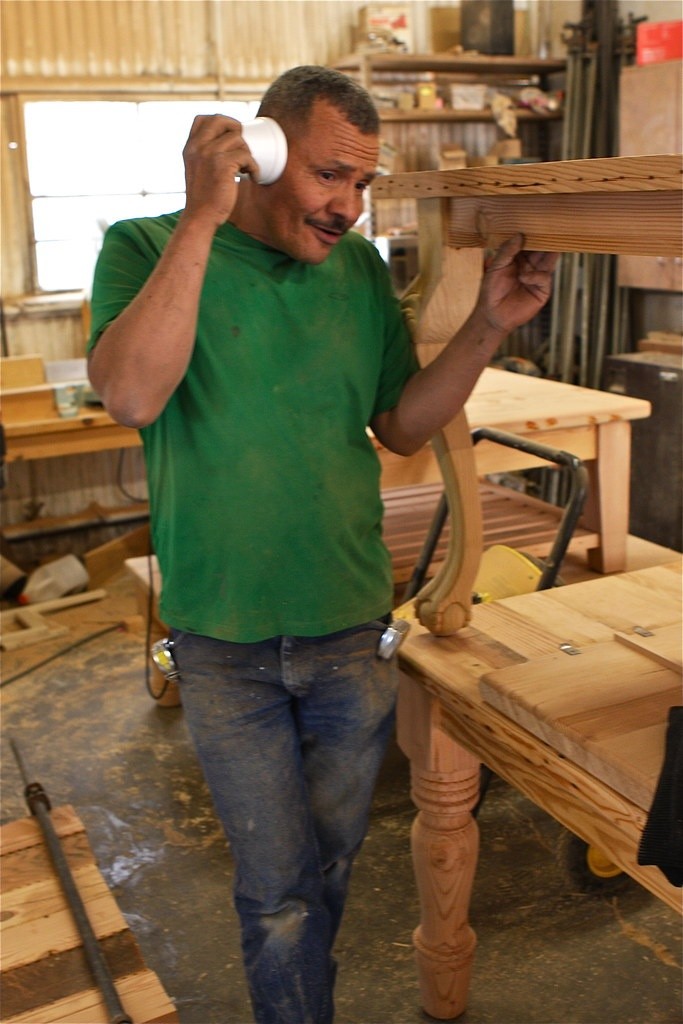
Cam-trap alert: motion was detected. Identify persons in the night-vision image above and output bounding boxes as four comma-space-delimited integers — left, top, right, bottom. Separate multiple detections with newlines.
85, 65, 559, 1024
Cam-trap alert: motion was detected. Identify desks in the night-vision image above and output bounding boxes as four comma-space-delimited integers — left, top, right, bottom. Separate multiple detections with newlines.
392, 565, 683, 1024
366, 155, 683, 636
348, 365, 652, 574
0, 352, 145, 464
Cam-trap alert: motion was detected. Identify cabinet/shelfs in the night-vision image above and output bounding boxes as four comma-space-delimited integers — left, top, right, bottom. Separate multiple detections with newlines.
326, 55, 568, 172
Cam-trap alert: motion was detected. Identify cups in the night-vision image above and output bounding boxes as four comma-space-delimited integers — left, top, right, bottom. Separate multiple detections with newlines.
238, 116, 288, 186
52, 385, 85, 419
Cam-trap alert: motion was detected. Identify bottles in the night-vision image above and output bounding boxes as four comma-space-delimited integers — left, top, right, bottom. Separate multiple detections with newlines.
19, 554, 90, 605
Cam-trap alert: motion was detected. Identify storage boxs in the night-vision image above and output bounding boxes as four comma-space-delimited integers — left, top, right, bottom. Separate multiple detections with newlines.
357, 3, 418, 58
